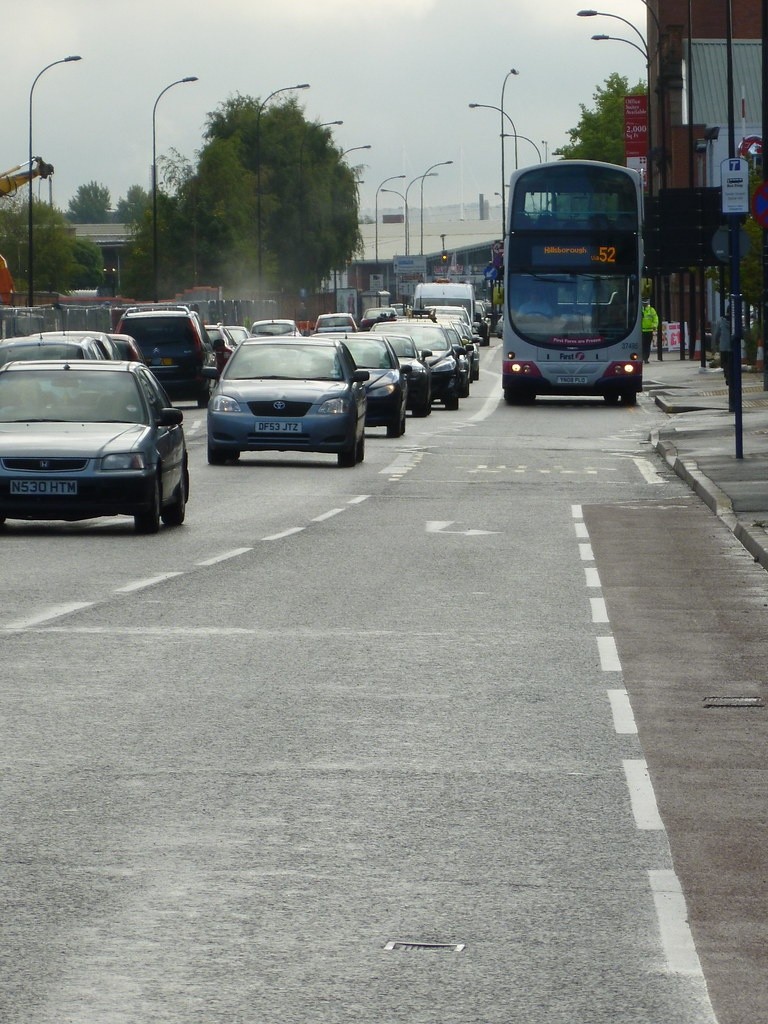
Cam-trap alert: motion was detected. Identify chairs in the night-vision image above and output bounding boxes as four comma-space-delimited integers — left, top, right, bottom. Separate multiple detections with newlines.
364, 347, 383, 367
11, 390, 139, 411
517, 208, 635, 229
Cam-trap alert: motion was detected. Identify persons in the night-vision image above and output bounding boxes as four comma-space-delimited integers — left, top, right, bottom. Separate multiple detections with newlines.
715, 304, 731, 385
641, 296, 658, 364
517, 287, 554, 320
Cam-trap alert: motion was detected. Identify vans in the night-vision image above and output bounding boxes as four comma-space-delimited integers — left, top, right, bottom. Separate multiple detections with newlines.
411, 283, 483, 334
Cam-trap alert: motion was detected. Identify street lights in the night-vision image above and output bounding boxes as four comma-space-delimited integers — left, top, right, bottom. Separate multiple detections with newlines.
26, 54, 83, 308
501, 68, 522, 240
499, 134, 543, 212
402, 173, 440, 256
150, 76, 199, 304
300, 120, 346, 300
374, 175, 408, 274
541, 140, 549, 162
332, 143, 372, 313
577, 10, 663, 363
468, 103, 518, 170
256, 84, 311, 301
420, 160, 454, 255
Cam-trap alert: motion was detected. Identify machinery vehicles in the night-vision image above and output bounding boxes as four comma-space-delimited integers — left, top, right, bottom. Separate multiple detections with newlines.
1, 154, 76, 308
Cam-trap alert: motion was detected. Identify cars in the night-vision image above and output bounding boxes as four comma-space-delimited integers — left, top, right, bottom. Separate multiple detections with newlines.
300, 333, 412, 439
0, 330, 149, 410
0, 358, 189, 535
201, 334, 371, 468
202, 299, 499, 419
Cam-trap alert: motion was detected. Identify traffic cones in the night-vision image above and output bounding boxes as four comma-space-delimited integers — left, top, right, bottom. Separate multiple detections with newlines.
739, 337, 751, 364
691, 329, 702, 361
754, 338, 765, 374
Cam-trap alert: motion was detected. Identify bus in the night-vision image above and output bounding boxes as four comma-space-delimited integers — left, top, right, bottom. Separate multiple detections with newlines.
492, 160, 655, 407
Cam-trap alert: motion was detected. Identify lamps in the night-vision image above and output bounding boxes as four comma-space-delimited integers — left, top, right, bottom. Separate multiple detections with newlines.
696, 124, 720, 154
645, 146, 660, 160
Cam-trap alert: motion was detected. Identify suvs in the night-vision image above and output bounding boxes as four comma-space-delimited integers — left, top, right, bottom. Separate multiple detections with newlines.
114, 303, 226, 410
